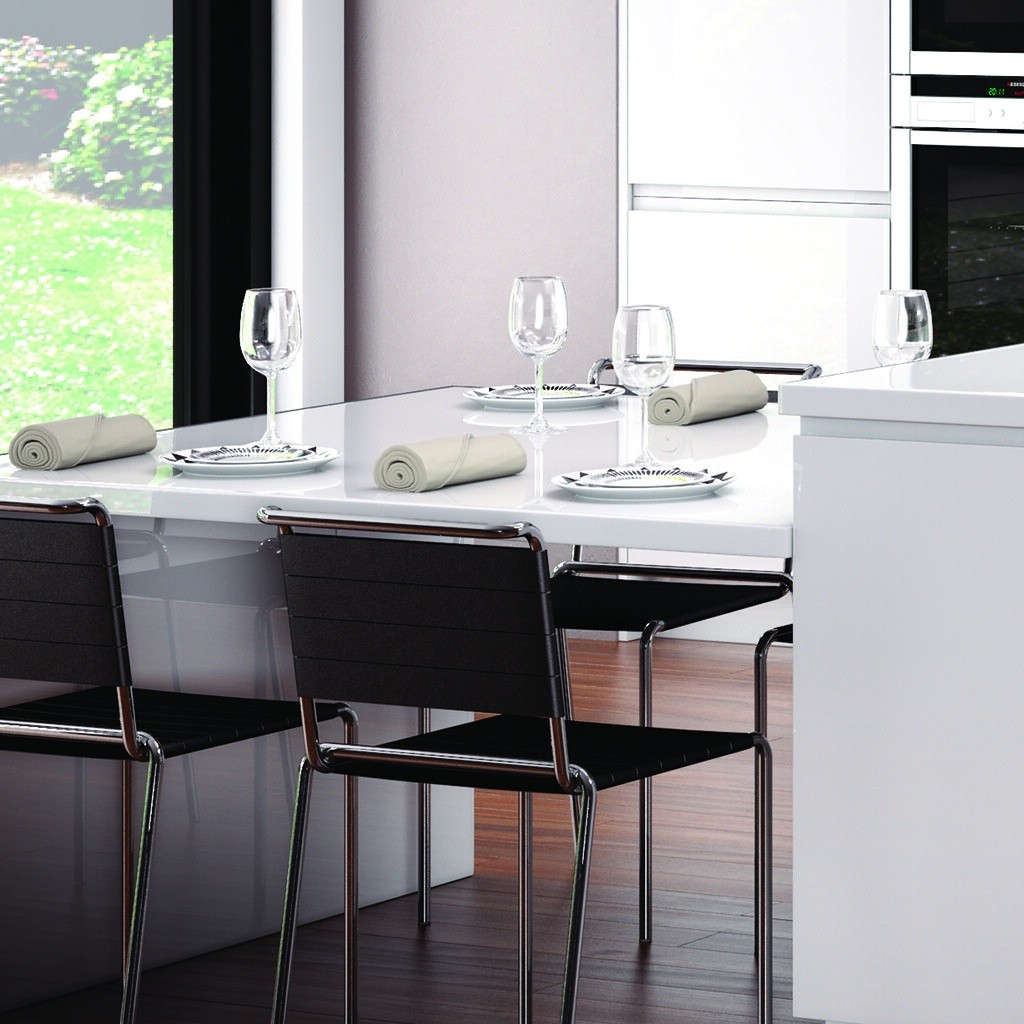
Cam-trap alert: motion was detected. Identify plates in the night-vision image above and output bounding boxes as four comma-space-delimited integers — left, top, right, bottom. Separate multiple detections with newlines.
464, 382, 625, 412
550, 467, 735, 502
163, 445, 338, 476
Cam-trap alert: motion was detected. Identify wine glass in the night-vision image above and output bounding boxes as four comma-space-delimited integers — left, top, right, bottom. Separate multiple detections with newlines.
510, 436, 567, 512
239, 287, 307, 445
508, 276, 570, 436
612, 305, 675, 465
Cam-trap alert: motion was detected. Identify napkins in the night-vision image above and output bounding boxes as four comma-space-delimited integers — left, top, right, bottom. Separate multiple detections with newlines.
10, 416, 157, 468
648, 370, 769, 427
374, 434, 527, 493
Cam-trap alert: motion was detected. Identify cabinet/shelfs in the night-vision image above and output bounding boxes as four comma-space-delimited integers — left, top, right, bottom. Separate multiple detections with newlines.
774, 344, 1024, 1024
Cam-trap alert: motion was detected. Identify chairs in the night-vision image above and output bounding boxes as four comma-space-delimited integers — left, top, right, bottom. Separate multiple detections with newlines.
0, 495, 360, 1024
544, 356, 821, 943
257, 505, 771, 1024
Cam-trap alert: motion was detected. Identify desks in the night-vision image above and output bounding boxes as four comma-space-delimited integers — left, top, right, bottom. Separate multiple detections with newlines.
0, 387, 797, 1011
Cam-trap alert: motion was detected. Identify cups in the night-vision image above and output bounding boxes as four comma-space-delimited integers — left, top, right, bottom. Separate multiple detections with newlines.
873, 289, 933, 366
